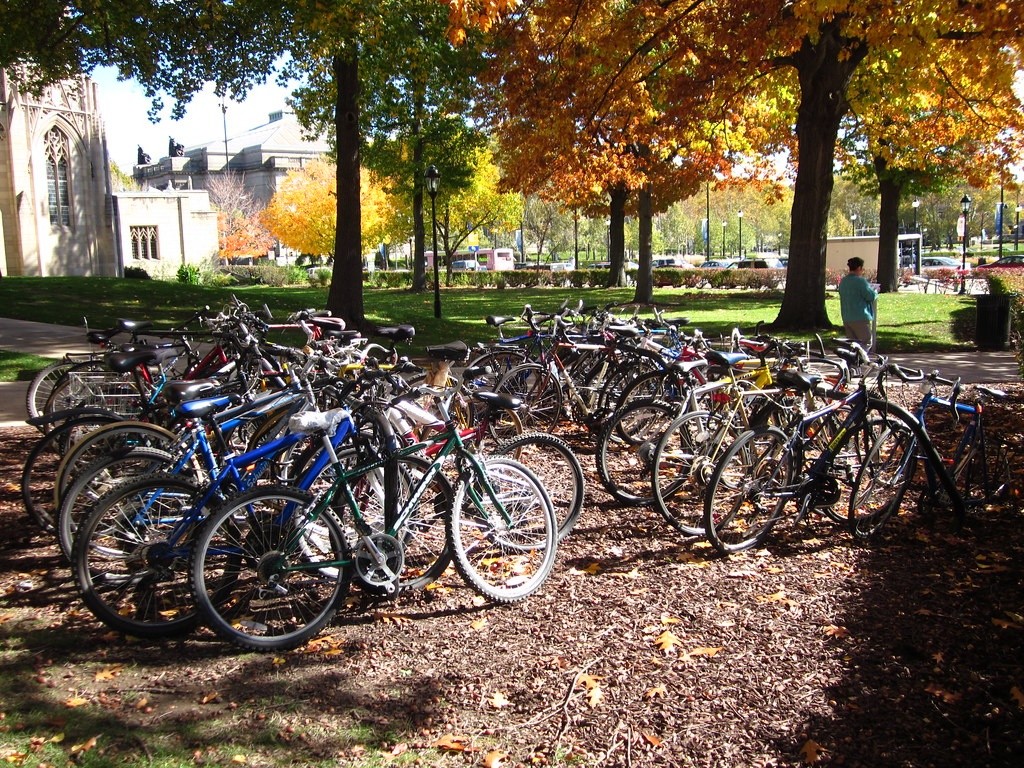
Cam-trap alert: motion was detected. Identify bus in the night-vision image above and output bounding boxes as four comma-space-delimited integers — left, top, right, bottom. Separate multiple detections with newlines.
423, 248, 515, 270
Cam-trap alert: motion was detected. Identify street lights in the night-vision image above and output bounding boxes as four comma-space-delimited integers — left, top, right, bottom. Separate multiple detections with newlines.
737, 210, 743, 260
606, 217, 611, 261
958, 194, 971, 295
1015, 205, 1021, 250
851, 213, 856, 236
981, 211, 991, 249
722, 221, 727, 256
424, 165, 443, 320
912, 195, 919, 233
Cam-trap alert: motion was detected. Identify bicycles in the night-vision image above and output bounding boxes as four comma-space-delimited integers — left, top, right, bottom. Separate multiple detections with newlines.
17, 295, 1024, 656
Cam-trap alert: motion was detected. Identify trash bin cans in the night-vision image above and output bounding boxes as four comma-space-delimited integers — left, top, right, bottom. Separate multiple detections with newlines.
974, 291, 1017, 351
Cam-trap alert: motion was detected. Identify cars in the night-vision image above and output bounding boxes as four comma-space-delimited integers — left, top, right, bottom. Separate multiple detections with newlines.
921, 258, 972, 279
587, 264, 610, 288
449, 261, 486, 271
307, 268, 333, 279
973, 256, 1024, 279
701, 260, 730, 269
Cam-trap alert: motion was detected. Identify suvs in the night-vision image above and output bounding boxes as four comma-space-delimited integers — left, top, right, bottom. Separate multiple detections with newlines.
728, 258, 784, 289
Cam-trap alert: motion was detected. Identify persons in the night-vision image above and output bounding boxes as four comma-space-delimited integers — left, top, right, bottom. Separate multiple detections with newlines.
838, 257, 881, 378
